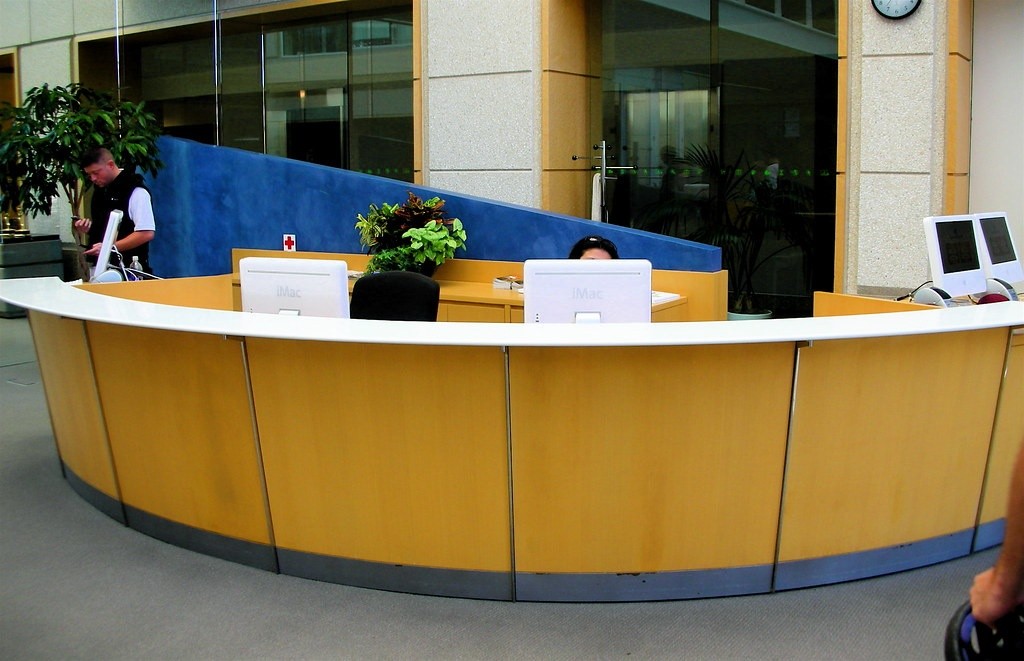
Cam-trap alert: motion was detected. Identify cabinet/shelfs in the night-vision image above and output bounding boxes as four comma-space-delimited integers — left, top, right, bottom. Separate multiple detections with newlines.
232, 272, 688, 323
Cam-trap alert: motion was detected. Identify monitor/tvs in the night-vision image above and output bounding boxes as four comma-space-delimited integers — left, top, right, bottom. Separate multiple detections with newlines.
524, 259, 652, 324
923, 212, 1024, 298
94, 209, 123, 277
239, 257, 350, 318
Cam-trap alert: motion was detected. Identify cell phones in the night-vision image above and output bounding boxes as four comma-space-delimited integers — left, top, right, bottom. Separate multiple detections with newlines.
71, 216, 81, 221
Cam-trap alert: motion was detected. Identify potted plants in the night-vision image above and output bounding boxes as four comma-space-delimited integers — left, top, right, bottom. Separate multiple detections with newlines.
354, 190, 467, 279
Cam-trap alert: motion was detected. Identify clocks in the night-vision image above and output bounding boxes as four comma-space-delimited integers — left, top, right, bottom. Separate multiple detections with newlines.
870, 1, 921, 21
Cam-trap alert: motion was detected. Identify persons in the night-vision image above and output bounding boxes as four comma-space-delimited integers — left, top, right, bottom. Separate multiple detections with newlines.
72, 148, 155, 280
969, 436, 1024, 624
568, 236, 618, 260
